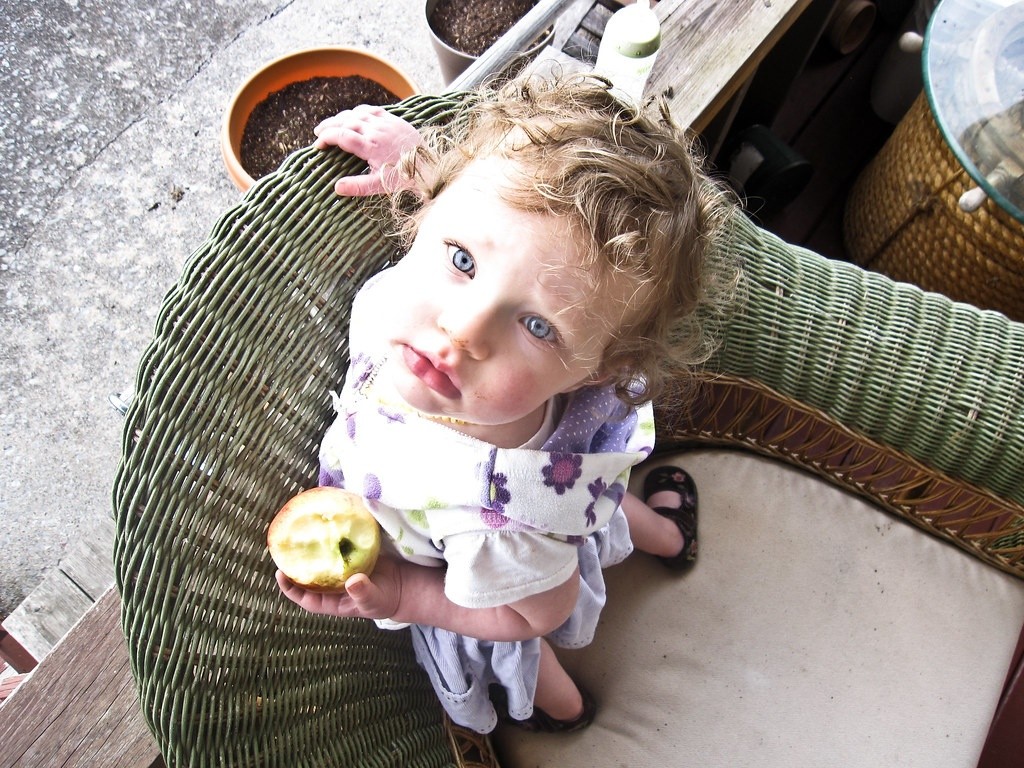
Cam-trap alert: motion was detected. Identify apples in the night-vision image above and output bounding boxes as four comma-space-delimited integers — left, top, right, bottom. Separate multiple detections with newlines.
267, 486, 379, 592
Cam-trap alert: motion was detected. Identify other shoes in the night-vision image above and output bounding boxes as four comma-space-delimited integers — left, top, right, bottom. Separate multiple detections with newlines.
489, 678, 596, 733
644, 465, 698, 571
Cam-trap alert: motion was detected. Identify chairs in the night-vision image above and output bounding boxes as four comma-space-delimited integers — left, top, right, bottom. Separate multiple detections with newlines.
114, 96, 1024, 767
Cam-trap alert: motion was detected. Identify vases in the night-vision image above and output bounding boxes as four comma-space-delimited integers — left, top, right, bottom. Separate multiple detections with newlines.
222, 47, 416, 192
425, 0, 555, 90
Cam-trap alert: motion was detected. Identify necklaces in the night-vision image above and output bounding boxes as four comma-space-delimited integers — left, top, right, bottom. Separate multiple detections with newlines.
353, 355, 477, 427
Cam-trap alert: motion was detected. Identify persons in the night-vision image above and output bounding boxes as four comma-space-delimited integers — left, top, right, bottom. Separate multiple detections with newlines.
314, 70, 738, 735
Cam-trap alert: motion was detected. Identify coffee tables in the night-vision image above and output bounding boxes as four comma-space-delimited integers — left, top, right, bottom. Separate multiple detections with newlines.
848, 0, 1023, 318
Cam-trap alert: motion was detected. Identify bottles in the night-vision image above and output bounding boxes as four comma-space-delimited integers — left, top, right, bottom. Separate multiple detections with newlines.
592, 0, 662, 109
